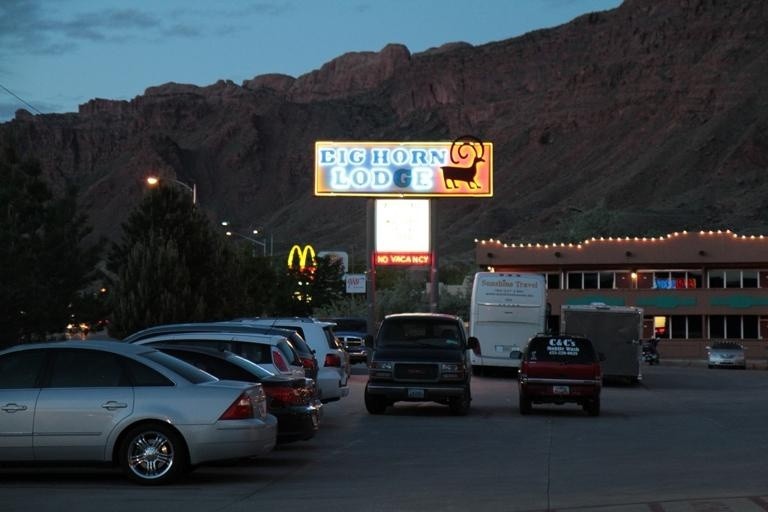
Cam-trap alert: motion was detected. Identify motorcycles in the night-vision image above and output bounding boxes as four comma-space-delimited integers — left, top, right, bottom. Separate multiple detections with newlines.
641, 336, 662, 364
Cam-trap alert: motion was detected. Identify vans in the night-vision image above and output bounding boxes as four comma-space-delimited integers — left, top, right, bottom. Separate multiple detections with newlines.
364, 313, 476, 415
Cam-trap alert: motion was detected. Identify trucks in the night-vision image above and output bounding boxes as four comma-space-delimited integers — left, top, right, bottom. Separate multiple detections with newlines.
560, 302, 644, 384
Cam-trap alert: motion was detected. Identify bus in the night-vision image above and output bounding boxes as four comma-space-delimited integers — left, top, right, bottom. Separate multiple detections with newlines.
468, 271, 552, 375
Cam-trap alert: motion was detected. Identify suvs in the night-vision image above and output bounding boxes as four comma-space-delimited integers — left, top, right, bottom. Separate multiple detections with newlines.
225, 319, 353, 406
123, 322, 321, 385
332, 320, 372, 365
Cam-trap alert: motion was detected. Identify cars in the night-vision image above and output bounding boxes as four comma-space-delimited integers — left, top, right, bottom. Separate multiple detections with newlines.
518, 332, 601, 415
133, 333, 306, 379
1, 338, 280, 485
142, 343, 324, 449
705, 341, 749, 370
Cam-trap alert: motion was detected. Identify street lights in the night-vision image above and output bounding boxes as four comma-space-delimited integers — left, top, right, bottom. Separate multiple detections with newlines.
147, 174, 197, 206
221, 221, 273, 258
